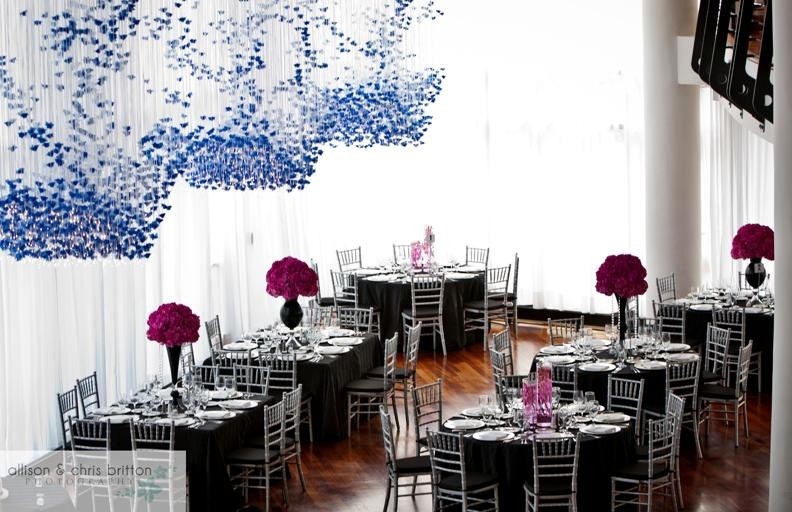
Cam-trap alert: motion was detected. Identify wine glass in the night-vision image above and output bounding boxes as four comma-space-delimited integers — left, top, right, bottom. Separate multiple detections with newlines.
242, 327, 321, 355
571, 325, 670, 367
116, 375, 237, 417
478, 387, 600, 439
383, 256, 459, 277
693, 278, 769, 307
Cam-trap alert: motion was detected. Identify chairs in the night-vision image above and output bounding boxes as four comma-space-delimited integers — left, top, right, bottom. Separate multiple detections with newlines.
611, 409, 679, 512
245, 384, 306, 504
57, 385, 79, 489
425, 425, 500, 512
129, 417, 190, 512
77, 370, 100, 417
378, 405, 431, 512
523, 431, 583, 511
638, 389, 686, 506
68, 415, 133, 512
409, 378, 442, 495
221, 392, 289, 512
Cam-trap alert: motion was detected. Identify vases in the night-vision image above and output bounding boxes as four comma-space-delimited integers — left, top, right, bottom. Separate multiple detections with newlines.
166, 345, 181, 387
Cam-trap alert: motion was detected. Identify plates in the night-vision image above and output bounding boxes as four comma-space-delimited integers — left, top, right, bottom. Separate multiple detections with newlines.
539, 339, 700, 372
222, 329, 363, 362
675, 288, 761, 313
349, 262, 483, 281
92, 390, 258, 427
444, 404, 631, 442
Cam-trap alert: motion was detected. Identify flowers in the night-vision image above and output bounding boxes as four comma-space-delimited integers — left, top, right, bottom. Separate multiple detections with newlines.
145, 302, 200, 348
